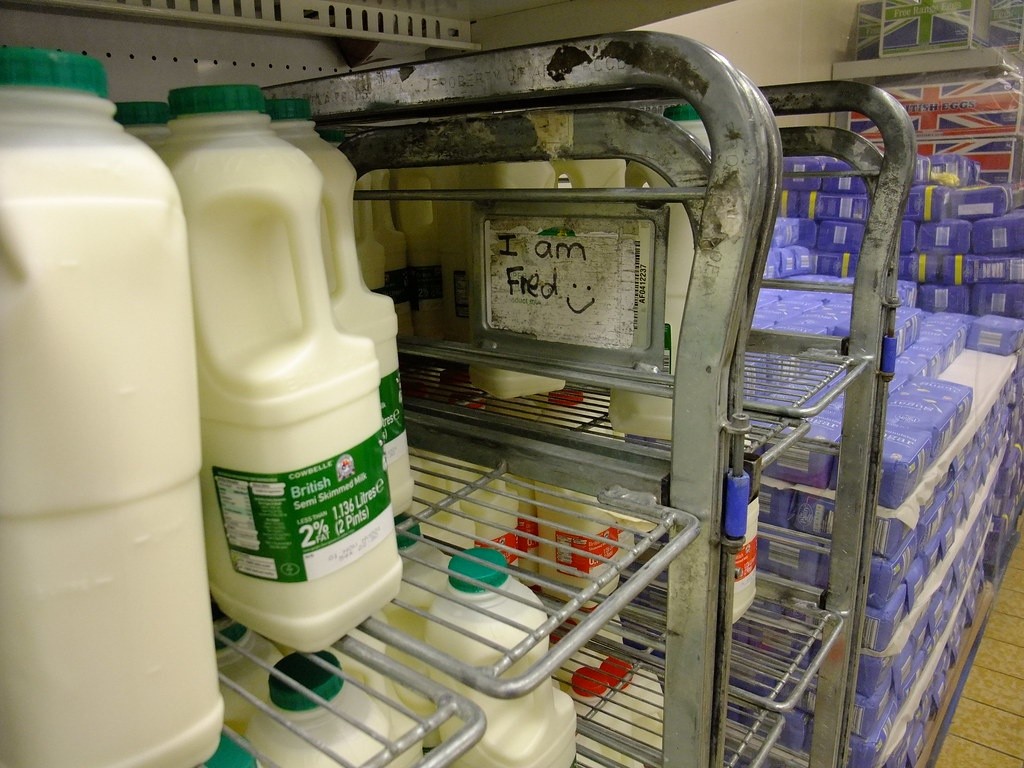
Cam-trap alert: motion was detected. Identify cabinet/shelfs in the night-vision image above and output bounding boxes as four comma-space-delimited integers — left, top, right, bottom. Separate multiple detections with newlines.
1, 32, 921, 768
